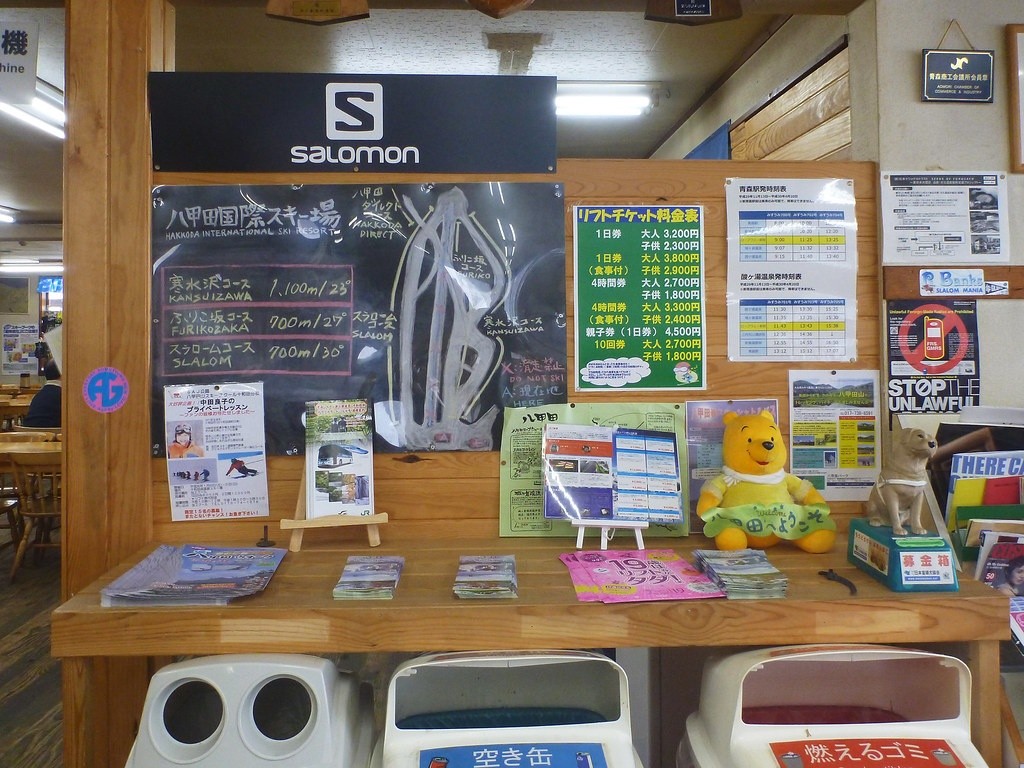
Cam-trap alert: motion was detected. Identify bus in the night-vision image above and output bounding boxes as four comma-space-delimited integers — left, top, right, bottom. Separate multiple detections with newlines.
318, 445, 353, 469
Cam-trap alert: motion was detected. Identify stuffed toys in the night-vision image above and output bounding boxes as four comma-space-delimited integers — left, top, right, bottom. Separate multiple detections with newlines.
696, 410, 836, 554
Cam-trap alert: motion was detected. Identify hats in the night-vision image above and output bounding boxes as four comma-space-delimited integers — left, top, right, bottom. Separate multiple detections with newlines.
175, 424, 191, 434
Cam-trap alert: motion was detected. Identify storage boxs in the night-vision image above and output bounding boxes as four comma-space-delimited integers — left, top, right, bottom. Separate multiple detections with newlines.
847, 518, 960, 593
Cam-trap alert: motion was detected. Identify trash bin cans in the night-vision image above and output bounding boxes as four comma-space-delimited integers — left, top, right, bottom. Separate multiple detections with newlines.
676, 643, 991, 767
371, 647, 646, 767
123, 650, 371, 767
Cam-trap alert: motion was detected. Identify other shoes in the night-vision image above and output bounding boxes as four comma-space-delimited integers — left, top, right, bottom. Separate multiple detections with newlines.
254, 470, 257, 475
245, 472, 248, 477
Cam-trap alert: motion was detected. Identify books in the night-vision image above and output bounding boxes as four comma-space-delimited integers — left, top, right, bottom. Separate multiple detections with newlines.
100, 543, 289, 607
934, 406, 1024, 657
691, 547, 788, 600
333, 556, 405, 600
452, 554, 519, 599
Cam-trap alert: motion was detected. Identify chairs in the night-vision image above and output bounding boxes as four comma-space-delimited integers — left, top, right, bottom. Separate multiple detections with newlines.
0, 383, 62, 586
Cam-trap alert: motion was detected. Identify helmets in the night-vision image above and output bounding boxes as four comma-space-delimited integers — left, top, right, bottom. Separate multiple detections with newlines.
231, 458, 237, 463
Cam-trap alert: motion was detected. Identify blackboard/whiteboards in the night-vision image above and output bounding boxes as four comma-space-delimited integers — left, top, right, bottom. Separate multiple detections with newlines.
148, 183, 568, 462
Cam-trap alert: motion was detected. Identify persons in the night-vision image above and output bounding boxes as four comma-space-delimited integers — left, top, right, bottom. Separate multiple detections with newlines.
174, 469, 210, 483
331, 416, 348, 432
226, 458, 258, 478
24, 359, 62, 428
996, 555, 1024, 596
169, 426, 204, 460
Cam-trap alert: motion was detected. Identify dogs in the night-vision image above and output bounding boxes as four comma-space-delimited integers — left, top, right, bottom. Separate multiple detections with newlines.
866, 427, 938, 535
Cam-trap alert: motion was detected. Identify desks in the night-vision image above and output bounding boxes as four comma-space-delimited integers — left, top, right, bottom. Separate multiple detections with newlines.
0, 442, 62, 472
0, 399, 33, 406
51, 532, 1011, 768
